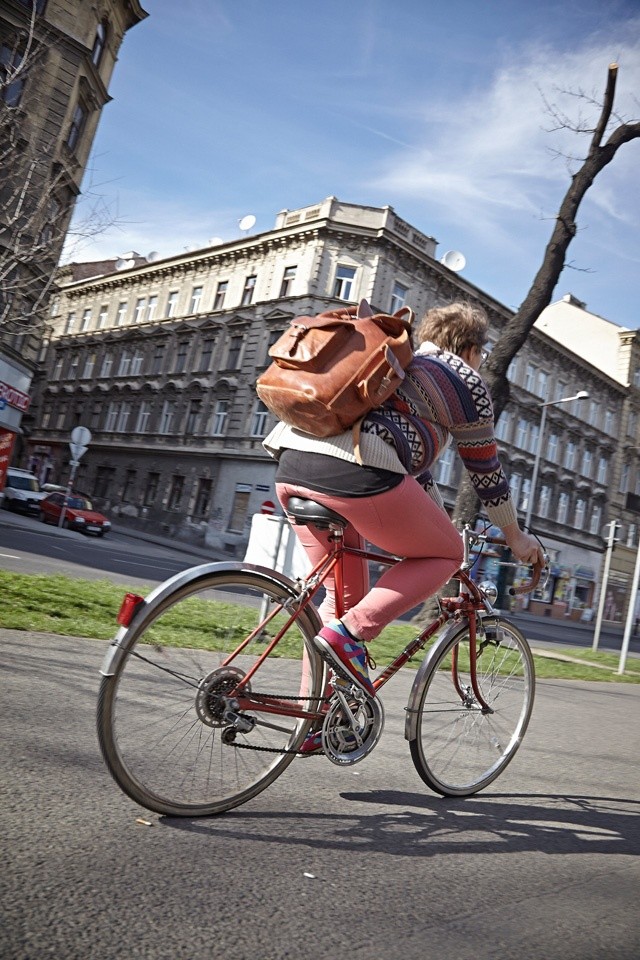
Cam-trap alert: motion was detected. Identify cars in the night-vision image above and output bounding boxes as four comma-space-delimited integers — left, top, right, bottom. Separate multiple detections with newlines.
41, 483, 94, 504
5, 467, 47, 515
39, 491, 111, 536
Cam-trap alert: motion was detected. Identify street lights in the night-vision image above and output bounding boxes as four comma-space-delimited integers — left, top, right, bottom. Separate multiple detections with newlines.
517, 390, 592, 534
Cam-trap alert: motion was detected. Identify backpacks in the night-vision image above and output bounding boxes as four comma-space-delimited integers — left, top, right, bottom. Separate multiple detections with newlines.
257, 297, 418, 439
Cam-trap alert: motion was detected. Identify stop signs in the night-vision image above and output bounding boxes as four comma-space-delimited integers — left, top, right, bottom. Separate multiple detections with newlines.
259, 501, 276, 515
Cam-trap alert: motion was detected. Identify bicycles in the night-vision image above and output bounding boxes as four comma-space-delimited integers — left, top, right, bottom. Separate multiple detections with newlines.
95, 497, 553, 817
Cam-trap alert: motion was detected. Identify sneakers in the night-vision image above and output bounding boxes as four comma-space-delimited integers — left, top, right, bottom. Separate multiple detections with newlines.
294, 721, 366, 759
313, 617, 377, 700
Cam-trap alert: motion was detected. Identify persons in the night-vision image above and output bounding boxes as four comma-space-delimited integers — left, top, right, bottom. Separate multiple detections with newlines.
262, 297, 544, 758
